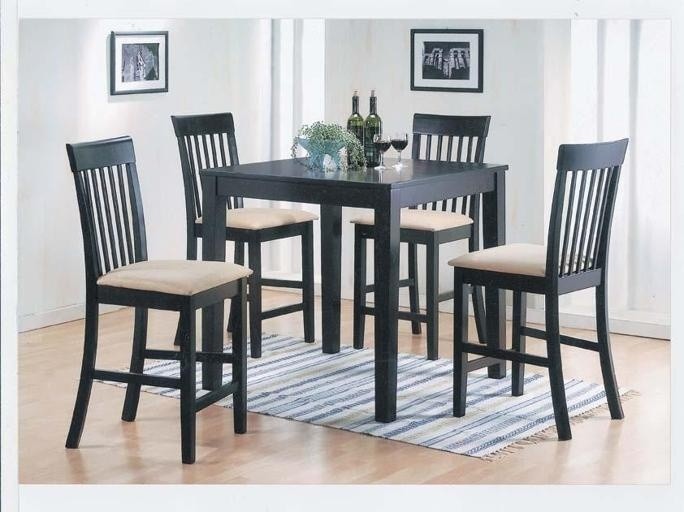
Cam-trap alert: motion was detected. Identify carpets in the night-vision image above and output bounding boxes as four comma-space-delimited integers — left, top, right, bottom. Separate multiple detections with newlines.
92, 332, 637, 463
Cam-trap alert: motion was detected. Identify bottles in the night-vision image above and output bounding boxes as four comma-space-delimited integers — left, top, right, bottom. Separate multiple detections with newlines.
346, 90, 364, 168
363, 90, 382, 167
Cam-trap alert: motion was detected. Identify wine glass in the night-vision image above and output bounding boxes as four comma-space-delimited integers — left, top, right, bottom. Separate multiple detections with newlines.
372, 133, 391, 171
389, 131, 409, 169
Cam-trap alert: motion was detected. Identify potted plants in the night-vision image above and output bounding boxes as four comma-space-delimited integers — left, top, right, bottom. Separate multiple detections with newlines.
291, 122, 368, 171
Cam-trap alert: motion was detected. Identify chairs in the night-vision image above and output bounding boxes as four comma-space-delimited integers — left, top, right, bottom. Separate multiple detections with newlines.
350, 112, 490, 360
170, 112, 314, 359
65, 134, 254, 464
449, 137, 629, 441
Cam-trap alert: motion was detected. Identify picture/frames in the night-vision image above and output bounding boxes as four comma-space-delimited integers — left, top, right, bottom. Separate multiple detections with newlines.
110, 31, 168, 95
410, 28, 485, 93
